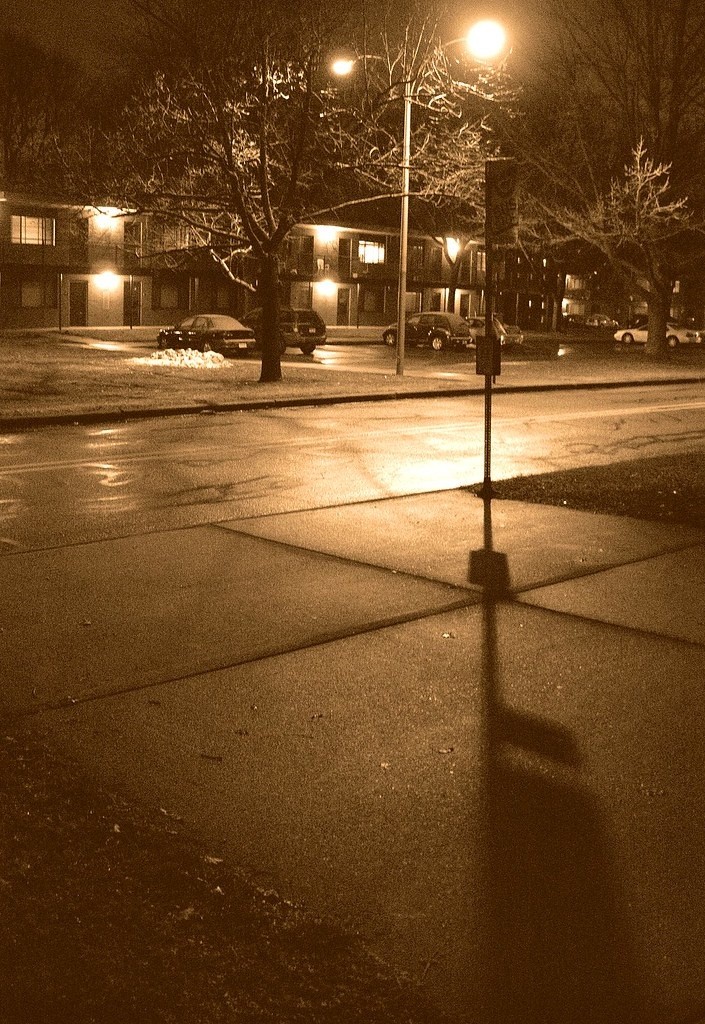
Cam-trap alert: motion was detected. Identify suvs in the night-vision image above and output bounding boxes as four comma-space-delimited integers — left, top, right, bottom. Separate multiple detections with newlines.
239, 306, 327, 352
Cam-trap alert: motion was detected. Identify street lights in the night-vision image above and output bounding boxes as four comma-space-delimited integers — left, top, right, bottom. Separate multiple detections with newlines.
330, 19, 508, 376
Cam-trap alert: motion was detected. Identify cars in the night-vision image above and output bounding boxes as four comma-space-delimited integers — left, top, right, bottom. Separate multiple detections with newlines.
562, 312, 619, 332
157, 314, 257, 356
464, 316, 525, 349
382, 313, 475, 353
616, 322, 702, 348
